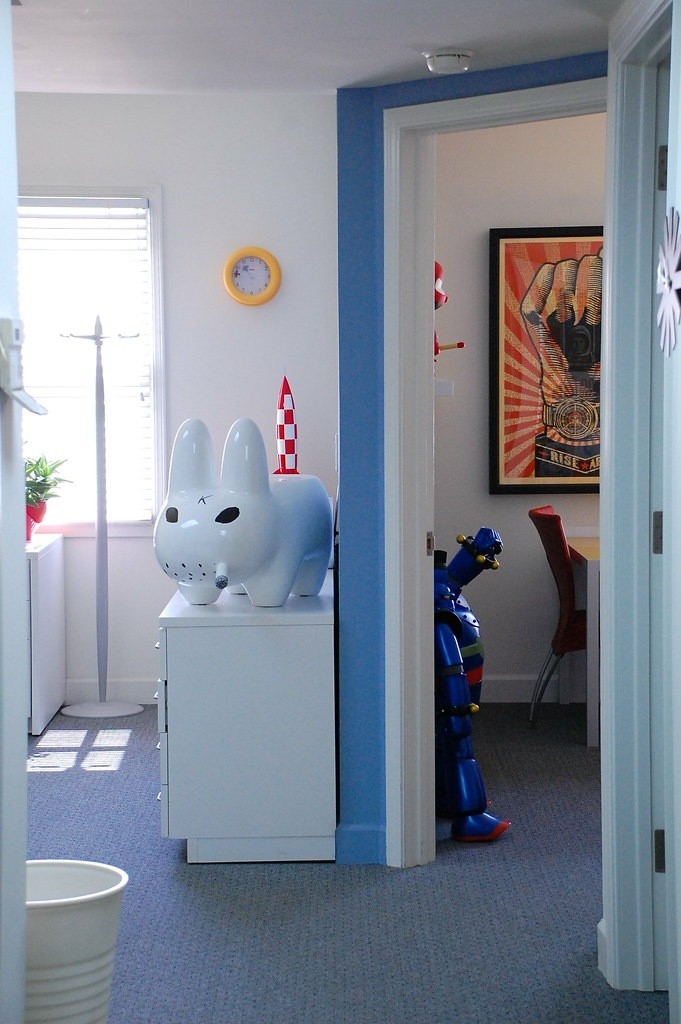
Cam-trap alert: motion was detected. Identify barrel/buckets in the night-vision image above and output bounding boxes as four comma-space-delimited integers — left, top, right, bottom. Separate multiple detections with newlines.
24, 856, 128, 1024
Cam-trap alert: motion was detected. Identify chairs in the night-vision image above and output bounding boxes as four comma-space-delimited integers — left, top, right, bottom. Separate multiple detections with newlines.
528, 504, 591, 724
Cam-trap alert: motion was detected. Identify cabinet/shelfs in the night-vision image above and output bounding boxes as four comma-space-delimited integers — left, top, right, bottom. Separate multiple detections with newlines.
25, 533, 66, 735
155, 567, 337, 864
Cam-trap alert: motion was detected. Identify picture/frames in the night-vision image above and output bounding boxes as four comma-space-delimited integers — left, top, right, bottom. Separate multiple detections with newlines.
488, 225, 606, 495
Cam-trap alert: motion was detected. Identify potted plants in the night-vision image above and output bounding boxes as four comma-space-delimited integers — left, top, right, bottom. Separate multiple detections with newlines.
25, 454, 74, 540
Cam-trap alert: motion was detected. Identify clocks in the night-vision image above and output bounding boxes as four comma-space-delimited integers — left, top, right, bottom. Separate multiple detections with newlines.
223, 247, 282, 304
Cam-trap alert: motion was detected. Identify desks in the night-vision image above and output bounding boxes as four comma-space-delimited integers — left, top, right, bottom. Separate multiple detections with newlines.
564, 536, 601, 748
